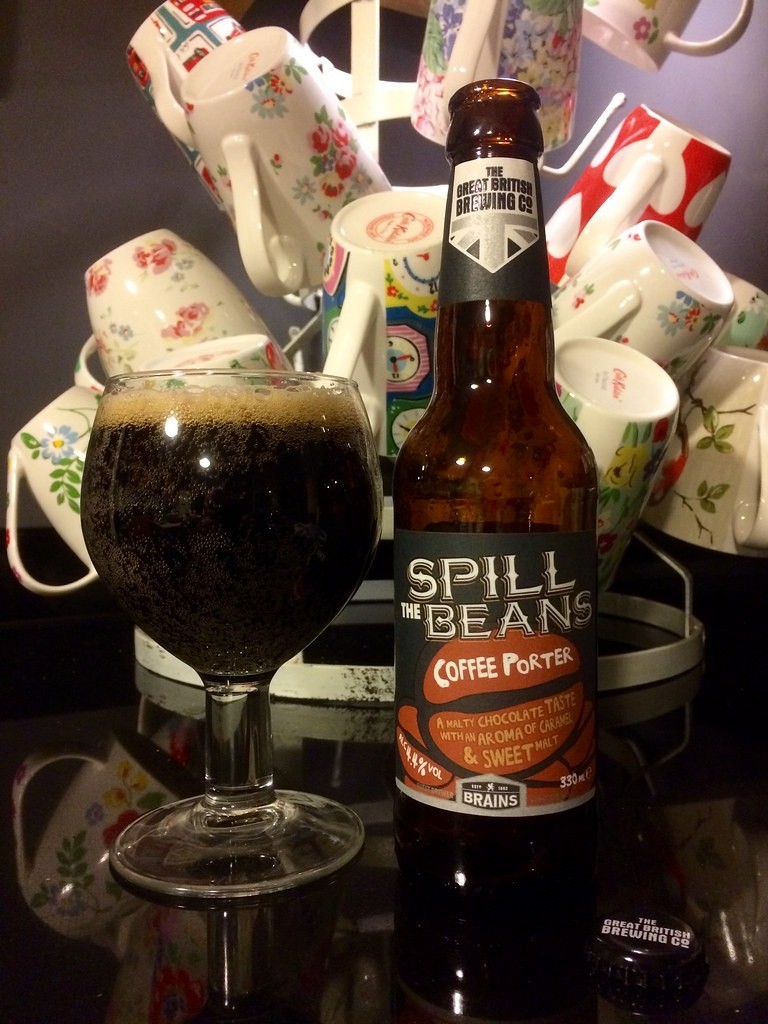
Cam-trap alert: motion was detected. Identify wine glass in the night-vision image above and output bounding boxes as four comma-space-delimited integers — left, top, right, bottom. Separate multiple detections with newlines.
80, 369, 366, 898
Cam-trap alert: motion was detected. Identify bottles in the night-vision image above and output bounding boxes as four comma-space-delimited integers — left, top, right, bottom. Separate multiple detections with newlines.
391, 80, 599, 901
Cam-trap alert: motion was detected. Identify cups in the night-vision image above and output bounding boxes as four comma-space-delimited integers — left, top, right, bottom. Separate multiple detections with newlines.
10, 726, 205, 940
410, 0, 754, 153
544, 219, 768, 599
5, 228, 296, 594
321, 189, 447, 459
544, 103, 733, 287
180, 26, 391, 298
125, 0, 248, 211
652, 785, 768, 1017
